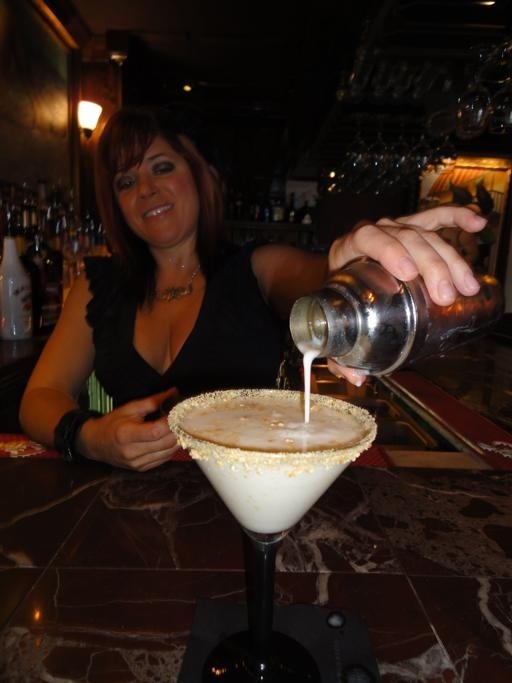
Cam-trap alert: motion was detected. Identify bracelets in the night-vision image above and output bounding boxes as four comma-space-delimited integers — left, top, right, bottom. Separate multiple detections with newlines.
53, 410, 107, 460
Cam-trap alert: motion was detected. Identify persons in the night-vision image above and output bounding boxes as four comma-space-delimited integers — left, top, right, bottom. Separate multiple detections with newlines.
17, 102, 491, 476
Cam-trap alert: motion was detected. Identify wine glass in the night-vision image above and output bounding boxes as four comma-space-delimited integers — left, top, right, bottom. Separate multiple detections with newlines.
172, 387, 377, 682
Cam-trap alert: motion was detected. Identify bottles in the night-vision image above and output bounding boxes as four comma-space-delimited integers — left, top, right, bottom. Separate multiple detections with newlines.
246, 175, 313, 225
289, 258, 501, 377
1, 179, 111, 342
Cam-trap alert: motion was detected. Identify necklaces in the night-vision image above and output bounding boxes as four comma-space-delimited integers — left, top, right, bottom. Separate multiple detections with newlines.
148, 260, 202, 299
